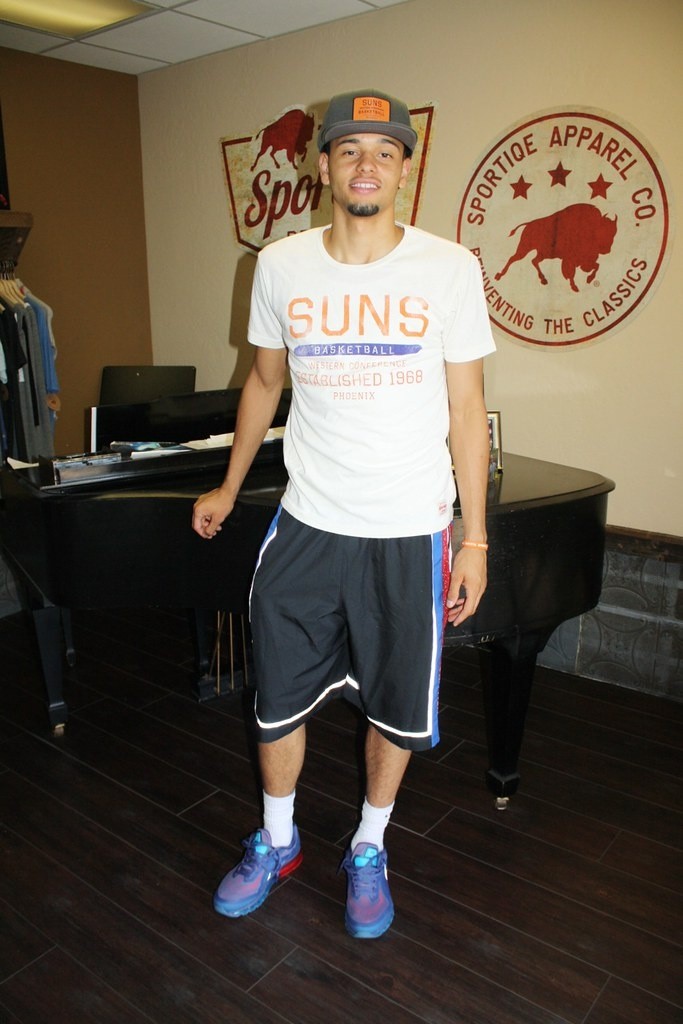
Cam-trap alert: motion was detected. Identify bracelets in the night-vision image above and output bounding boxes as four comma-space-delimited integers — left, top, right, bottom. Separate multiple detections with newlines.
460, 540, 489, 550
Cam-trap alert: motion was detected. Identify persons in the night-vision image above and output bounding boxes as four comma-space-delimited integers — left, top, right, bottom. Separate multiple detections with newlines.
193, 91, 497, 935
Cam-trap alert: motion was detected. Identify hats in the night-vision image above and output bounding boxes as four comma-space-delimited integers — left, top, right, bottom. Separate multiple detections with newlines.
316, 88, 419, 155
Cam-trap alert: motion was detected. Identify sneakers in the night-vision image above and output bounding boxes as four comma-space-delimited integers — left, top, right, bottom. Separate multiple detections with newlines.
344, 842, 395, 939
213, 824, 303, 916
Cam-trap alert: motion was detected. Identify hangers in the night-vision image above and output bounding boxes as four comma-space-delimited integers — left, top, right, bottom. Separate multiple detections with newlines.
0, 259, 26, 315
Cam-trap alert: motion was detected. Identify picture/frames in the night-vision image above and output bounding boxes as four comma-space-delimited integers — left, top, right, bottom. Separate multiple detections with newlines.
452, 411, 503, 470
